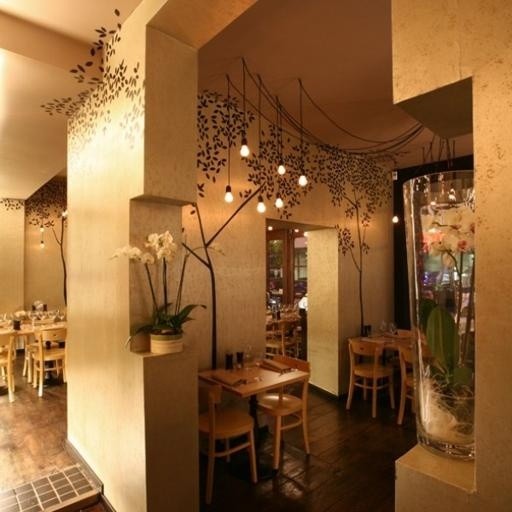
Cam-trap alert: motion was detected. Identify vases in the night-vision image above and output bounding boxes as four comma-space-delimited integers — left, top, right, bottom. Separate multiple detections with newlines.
403, 169, 476, 462
149, 332, 183, 355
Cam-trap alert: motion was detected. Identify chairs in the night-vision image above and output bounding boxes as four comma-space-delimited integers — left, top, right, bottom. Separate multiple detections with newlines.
0, 305, 69, 402
346, 329, 417, 426
198, 354, 311, 504
266, 319, 300, 359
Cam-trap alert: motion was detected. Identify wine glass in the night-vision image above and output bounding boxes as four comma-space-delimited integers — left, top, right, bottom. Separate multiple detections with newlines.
0, 304, 65, 330
379, 320, 397, 339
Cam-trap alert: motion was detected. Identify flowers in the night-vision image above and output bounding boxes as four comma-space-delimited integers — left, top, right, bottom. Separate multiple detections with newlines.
108, 229, 227, 348
417, 200, 476, 441
